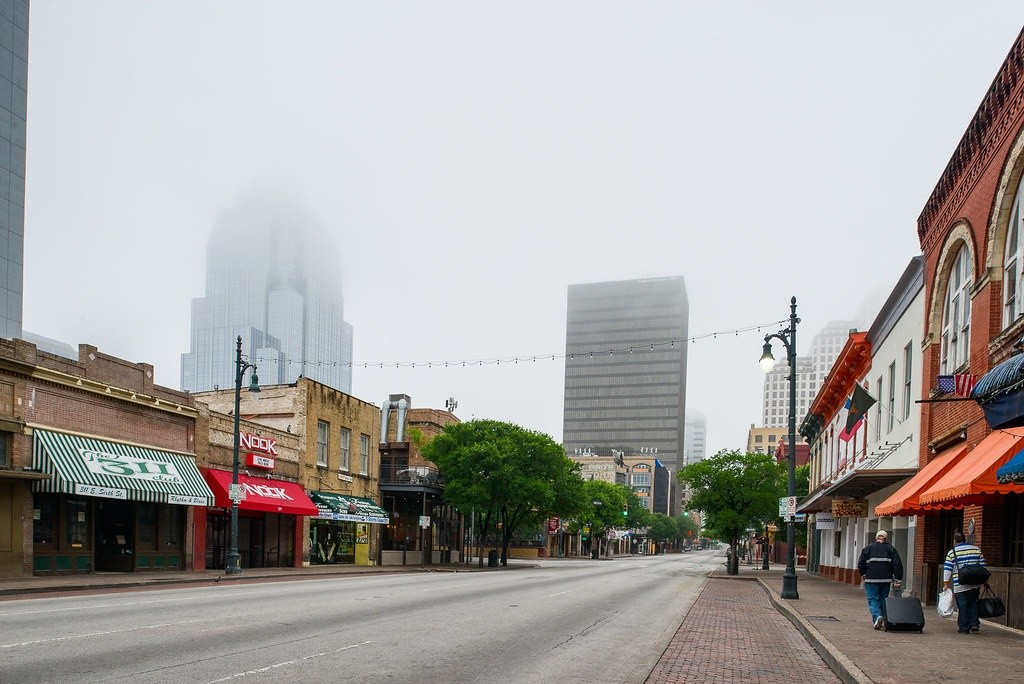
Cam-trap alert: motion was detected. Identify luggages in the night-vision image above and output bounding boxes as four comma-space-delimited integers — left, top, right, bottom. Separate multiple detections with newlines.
885, 583, 925, 633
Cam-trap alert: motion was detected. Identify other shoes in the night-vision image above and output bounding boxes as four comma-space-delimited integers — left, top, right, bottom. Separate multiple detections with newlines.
957, 630, 970, 634
874, 616, 884, 630
971, 626, 979, 632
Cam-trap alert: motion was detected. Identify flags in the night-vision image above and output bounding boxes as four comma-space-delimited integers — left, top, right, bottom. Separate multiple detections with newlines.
938, 375, 977, 398
838, 383, 877, 442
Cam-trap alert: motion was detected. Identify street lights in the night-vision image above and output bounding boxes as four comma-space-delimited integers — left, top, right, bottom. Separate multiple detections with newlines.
758, 294, 802, 600
225, 335, 261, 574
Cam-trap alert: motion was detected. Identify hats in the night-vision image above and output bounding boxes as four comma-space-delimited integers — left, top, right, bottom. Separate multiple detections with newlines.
875, 530, 888, 540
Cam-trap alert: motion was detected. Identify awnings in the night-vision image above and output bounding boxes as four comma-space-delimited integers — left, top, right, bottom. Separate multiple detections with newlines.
874, 427, 1024, 516
970, 352, 1024, 429
30, 428, 389, 525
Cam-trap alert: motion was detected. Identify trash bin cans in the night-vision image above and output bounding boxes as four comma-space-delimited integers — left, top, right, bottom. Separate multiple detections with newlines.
727, 555, 738, 575
488, 550, 499, 567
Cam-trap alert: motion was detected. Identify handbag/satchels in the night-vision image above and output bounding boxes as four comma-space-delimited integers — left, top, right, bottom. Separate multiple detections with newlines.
937, 587, 954, 618
977, 587, 1005, 618
953, 548, 991, 585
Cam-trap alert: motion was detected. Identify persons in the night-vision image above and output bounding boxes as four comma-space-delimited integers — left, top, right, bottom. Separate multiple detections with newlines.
943, 532, 986, 633
858, 530, 903, 629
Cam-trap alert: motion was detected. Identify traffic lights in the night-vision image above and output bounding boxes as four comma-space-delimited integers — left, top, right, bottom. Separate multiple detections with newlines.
623, 503, 628, 516
